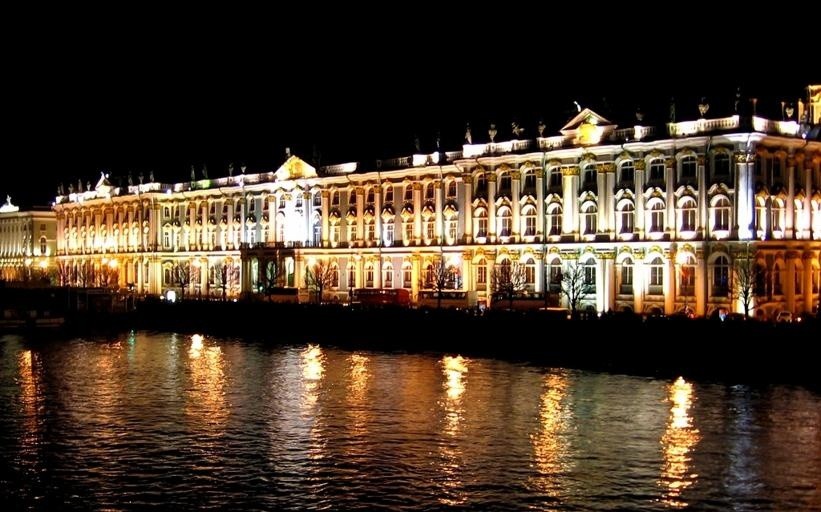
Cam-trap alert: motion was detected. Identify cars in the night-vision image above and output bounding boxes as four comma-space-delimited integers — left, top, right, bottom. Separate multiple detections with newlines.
776, 311, 792, 323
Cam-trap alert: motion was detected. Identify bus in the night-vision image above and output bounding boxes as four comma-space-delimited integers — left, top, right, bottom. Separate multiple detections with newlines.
354, 287, 477, 310
262, 286, 309, 304
488, 290, 561, 313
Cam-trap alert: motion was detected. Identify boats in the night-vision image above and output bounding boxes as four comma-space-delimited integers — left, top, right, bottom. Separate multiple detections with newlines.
0, 316, 64, 329
